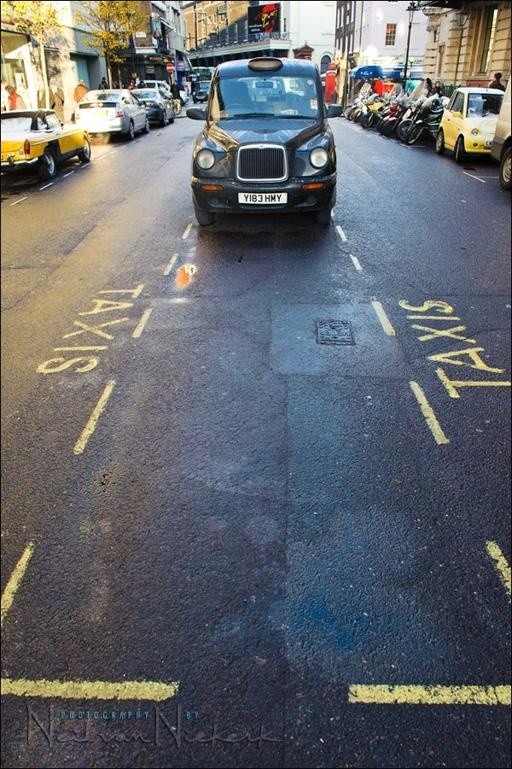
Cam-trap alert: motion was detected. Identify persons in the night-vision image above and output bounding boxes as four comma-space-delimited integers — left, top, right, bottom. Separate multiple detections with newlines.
73, 79, 89, 104
170, 80, 184, 114
36, 79, 64, 122
98, 76, 146, 90
489, 72, 505, 91
1, 84, 26, 112
422, 78, 443, 97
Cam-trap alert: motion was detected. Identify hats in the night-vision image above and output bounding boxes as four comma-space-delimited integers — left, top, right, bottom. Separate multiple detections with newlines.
78, 80, 85, 85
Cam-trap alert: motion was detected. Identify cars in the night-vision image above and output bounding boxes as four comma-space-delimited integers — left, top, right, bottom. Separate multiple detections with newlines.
185, 55, 342, 229
436, 87, 505, 160
1, 110, 92, 182
73, 80, 213, 141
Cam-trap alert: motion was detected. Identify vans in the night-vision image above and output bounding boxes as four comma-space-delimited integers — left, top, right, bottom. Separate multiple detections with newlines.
490, 75, 512, 189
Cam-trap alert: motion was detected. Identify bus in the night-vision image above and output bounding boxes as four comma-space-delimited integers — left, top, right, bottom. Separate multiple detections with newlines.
191, 67, 211, 80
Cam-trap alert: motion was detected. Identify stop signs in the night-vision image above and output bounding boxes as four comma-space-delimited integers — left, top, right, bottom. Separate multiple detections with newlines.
166, 64, 174, 74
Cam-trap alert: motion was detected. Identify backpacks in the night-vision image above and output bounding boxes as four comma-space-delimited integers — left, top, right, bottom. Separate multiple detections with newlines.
100, 83, 105, 90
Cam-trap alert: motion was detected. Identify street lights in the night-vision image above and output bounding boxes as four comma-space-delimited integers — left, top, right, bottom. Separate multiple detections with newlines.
399, 2, 418, 90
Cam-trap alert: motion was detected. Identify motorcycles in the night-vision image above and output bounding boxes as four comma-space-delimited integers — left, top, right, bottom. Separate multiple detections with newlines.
344, 82, 452, 147
